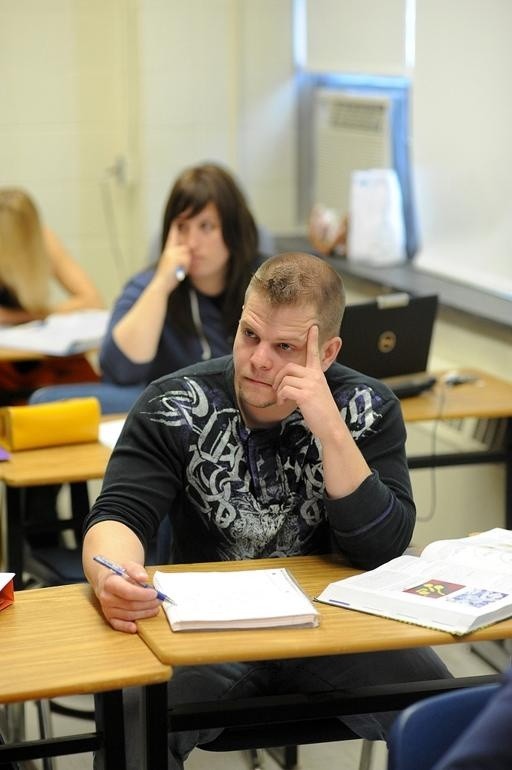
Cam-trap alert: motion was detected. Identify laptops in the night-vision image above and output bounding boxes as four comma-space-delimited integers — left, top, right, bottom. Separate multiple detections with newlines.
336, 293, 440, 380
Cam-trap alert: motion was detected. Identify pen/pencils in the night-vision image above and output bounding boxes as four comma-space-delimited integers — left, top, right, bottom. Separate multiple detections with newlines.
93, 555, 177, 606
176, 266, 186, 281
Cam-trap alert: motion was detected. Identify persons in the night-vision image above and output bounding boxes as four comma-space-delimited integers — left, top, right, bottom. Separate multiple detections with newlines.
101, 161, 271, 389
0, 190, 101, 395
81, 249, 458, 769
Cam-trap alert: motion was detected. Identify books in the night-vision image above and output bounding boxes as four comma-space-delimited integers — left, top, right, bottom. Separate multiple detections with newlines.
3, 308, 119, 361
152, 566, 322, 635
97, 415, 128, 450
320, 524, 512, 635
